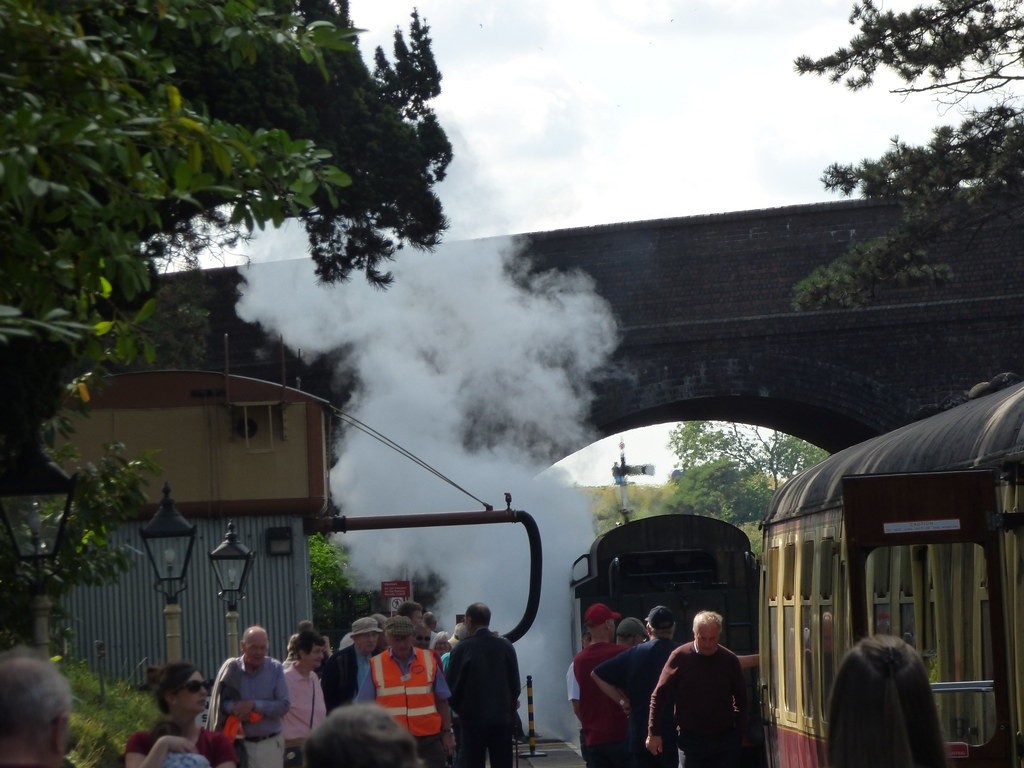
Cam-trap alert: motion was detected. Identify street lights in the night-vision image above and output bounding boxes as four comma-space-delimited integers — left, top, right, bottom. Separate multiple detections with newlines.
207, 519, 255, 657
138, 480, 198, 663
0, 412, 81, 655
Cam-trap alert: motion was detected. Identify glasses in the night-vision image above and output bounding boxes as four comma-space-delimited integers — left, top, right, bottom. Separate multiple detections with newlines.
178, 679, 211, 693
68, 695, 85, 711
415, 636, 431, 642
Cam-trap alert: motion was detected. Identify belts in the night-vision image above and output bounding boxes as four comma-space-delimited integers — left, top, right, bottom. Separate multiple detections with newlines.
245, 729, 282, 743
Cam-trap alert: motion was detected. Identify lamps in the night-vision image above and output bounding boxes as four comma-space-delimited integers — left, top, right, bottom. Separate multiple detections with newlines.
267, 526, 293, 556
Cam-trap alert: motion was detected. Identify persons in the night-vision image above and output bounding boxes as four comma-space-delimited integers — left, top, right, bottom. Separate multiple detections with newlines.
568, 603, 685, 768
307, 704, 417, 768
125, 662, 235, 768
206, 626, 291, 768
827, 635, 948, 768
0, 656, 75, 768
352, 616, 456, 767
283, 602, 470, 768
445, 602, 522, 768
645, 611, 753, 767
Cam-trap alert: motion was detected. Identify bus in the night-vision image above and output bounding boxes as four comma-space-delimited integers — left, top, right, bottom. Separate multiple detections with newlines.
759, 375, 1024, 768
568, 513, 764, 754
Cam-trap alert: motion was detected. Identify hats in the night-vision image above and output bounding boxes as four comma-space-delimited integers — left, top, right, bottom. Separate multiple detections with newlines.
384, 615, 416, 636
349, 617, 383, 636
617, 617, 649, 643
448, 622, 466, 647
643, 606, 675, 629
585, 603, 620, 628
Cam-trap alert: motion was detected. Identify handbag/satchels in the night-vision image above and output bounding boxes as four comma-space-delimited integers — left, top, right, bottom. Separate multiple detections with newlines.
284, 746, 303, 767
